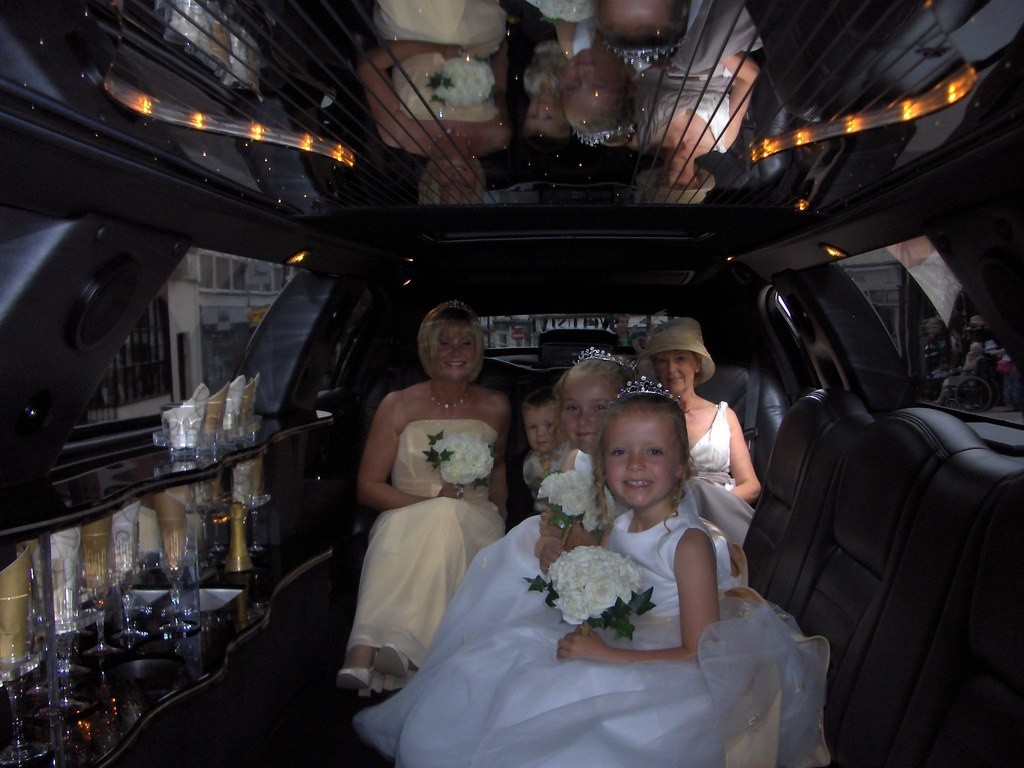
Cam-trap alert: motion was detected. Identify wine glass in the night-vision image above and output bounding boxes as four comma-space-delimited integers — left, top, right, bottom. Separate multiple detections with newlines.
0, 464, 271, 768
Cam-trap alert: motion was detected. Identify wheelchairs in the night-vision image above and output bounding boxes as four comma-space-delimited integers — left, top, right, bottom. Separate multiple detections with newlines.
935, 365, 995, 418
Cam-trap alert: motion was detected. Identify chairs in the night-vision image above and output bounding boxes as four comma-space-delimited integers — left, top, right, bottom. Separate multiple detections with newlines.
691, 364, 1024, 768
705, 0, 1024, 189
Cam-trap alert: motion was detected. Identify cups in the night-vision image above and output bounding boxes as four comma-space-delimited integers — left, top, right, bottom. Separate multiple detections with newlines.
152, 396, 258, 462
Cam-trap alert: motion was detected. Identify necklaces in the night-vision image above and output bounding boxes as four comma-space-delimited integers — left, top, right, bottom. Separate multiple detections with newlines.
426, 382, 469, 409
421, 129, 471, 157
684, 397, 699, 413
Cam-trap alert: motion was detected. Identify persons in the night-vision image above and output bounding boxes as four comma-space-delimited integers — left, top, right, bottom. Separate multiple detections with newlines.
915, 315, 1024, 413
351, 316, 835, 768
357, 0, 765, 206
336, 301, 508, 698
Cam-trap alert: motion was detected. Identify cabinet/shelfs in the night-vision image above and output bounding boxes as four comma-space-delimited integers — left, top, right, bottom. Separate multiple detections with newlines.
0, 407, 335, 768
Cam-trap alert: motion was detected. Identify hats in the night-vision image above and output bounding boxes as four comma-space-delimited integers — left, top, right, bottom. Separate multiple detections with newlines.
635, 317, 716, 386
636, 164, 717, 206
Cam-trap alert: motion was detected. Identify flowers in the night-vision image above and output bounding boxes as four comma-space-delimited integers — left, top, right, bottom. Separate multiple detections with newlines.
427, 56, 496, 110
522, 543, 656, 642
526, 0, 598, 27
423, 429, 498, 492
536, 470, 616, 548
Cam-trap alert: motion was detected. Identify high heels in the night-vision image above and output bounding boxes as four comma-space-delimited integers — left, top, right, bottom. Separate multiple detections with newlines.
336, 666, 375, 701
373, 642, 409, 691
